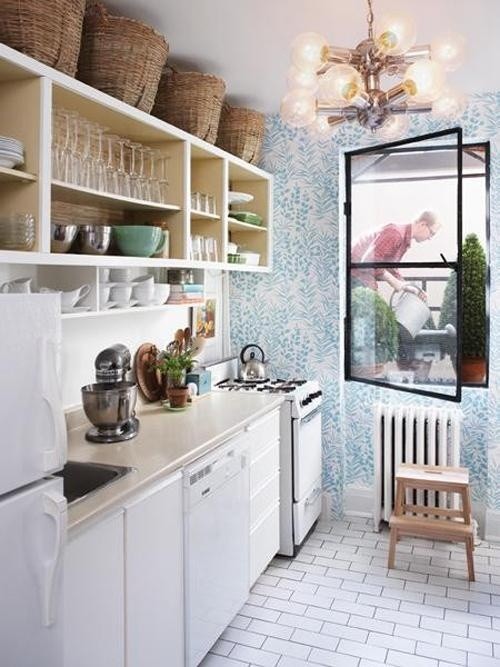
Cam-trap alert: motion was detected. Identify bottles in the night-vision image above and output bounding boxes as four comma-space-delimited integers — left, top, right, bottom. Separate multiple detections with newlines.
191, 234, 222, 261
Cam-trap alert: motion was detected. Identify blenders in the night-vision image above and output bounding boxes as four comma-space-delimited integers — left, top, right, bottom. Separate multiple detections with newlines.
81, 344, 139, 443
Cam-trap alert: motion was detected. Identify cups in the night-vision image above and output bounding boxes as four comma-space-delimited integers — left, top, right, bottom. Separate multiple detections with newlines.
99, 268, 153, 309
191, 192, 217, 215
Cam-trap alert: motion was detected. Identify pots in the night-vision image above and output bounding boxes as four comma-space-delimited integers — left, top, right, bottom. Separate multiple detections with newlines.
168, 270, 195, 285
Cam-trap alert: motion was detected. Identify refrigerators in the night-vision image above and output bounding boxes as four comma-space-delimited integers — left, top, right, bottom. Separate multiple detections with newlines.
0, 292, 68, 667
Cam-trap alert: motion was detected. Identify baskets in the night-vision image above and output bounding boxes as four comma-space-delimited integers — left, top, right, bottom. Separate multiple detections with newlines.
75, 0, 169, 117
158, 70, 227, 148
216, 104, 267, 169
1, 0, 89, 79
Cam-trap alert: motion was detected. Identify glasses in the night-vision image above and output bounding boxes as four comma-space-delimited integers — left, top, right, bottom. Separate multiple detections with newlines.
425, 223, 436, 237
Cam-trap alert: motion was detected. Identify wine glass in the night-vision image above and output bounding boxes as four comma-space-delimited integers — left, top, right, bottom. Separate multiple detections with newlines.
52, 106, 171, 204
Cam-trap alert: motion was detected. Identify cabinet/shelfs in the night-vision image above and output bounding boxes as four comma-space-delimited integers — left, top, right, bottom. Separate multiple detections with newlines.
0, 44, 275, 274
245, 410, 281, 593
1, 267, 195, 413
60, 471, 184, 667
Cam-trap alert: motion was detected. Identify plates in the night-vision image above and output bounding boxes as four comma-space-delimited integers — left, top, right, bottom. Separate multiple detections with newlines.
136, 343, 167, 401
164, 403, 194, 411
0, 214, 35, 250
61, 307, 91, 313
0, 136, 24, 168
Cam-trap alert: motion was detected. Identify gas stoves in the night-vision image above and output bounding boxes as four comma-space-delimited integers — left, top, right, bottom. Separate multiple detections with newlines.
213, 377, 323, 419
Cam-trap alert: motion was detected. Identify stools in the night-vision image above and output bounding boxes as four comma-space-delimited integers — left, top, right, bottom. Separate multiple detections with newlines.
389, 462, 475, 584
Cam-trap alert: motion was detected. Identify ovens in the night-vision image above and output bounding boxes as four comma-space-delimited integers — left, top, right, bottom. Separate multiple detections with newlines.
277, 401, 323, 557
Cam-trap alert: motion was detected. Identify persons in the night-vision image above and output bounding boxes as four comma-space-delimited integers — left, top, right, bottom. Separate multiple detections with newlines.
350, 211, 440, 302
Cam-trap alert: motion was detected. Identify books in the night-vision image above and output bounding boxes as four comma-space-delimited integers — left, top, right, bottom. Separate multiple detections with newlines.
166, 284, 204, 303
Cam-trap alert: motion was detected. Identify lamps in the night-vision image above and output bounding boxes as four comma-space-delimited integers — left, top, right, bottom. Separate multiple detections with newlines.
281, 0, 466, 139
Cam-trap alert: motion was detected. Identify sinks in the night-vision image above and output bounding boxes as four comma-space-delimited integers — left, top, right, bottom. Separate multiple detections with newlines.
63, 461, 138, 510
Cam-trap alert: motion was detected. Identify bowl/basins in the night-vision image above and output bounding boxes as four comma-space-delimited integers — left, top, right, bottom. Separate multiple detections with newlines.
51, 223, 112, 254
133, 275, 170, 306
229, 254, 240, 263
112, 225, 167, 257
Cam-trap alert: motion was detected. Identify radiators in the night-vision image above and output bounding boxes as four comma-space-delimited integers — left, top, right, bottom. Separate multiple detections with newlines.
373, 403, 480, 544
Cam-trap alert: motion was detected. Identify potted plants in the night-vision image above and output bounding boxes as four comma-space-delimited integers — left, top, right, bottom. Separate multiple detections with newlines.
441, 228, 491, 386
149, 344, 201, 409
349, 284, 396, 379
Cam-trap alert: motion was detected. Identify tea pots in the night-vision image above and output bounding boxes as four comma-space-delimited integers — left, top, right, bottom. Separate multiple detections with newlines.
239, 344, 271, 382
